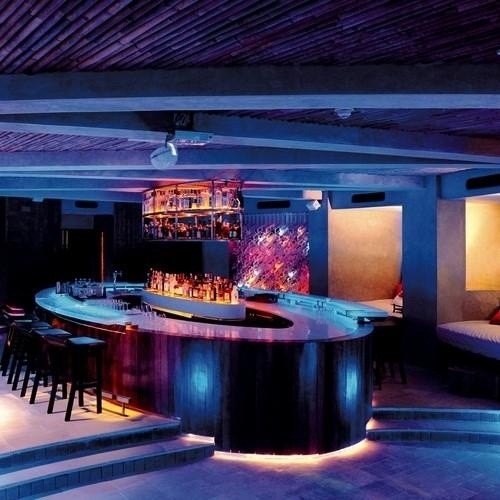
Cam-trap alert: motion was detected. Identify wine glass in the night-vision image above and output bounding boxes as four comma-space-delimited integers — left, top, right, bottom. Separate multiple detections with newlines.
101, 298, 156, 322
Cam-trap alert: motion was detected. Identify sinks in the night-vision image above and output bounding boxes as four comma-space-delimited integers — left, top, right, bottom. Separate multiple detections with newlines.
118, 288, 142, 292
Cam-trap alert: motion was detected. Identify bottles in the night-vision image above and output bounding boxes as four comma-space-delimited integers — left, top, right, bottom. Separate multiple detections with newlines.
144, 269, 241, 304
55, 277, 103, 299
142, 187, 239, 239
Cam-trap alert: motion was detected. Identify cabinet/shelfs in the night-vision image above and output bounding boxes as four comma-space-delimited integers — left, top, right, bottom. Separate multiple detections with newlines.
141, 181, 243, 243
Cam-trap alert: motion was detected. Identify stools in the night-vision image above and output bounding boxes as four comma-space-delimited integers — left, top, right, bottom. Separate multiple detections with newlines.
364, 311, 408, 394
0, 302, 107, 422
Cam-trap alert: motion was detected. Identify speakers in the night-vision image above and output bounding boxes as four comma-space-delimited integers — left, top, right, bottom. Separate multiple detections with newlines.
306, 200, 320, 210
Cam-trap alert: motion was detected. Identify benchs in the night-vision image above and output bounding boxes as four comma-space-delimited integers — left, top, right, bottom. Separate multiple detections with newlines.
352, 299, 403, 326
438, 320, 500, 362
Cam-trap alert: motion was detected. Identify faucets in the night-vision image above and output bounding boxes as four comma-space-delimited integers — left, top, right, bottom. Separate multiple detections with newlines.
113, 270, 122, 285
